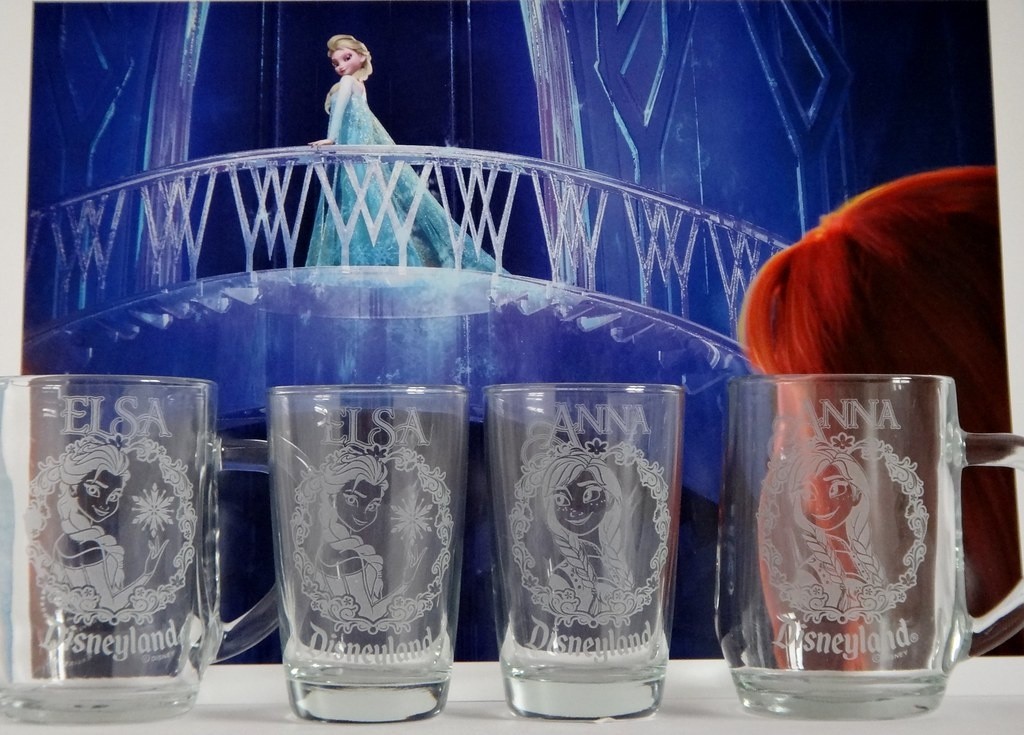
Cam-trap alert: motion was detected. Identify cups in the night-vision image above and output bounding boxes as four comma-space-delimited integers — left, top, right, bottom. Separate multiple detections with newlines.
0, 374, 323, 723
713, 371, 1024, 720
266, 384, 469, 725
485, 381, 685, 722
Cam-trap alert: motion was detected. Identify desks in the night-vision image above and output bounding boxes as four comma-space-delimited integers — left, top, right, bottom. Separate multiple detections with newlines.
0, 657, 1024, 735
